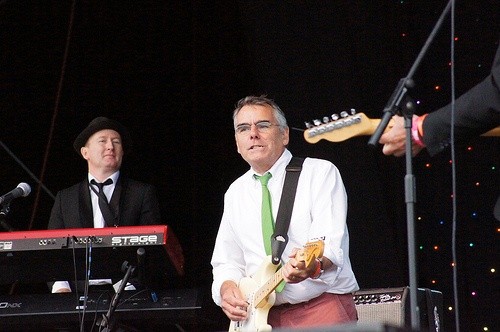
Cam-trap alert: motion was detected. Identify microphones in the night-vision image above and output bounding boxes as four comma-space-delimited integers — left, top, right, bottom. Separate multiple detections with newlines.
0, 181, 32, 204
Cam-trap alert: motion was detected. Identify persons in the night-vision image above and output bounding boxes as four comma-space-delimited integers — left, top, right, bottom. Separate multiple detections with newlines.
210, 96, 360, 330
377, 45, 500, 159
43, 115, 162, 294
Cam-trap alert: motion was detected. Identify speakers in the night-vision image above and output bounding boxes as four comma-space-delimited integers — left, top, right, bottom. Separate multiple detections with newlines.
350, 286, 445, 332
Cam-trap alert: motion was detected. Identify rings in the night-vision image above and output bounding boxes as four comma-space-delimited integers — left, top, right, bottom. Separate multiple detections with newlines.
292, 276, 297, 281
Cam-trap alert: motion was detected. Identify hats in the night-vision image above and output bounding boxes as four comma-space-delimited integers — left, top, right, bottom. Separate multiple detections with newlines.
73, 116, 128, 152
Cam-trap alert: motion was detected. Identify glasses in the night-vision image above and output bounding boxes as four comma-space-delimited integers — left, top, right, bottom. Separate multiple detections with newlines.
235, 121, 283, 135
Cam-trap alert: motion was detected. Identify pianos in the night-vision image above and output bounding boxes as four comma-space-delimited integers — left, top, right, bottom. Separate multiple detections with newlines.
0, 223, 223, 332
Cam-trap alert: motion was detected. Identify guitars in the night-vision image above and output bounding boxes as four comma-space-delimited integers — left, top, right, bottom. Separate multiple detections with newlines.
228, 235, 325, 332
304, 109, 500, 143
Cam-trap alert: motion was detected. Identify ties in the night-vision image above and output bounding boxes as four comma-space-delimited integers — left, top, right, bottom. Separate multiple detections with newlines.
253, 171, 286, 294
90, 178, 118, 228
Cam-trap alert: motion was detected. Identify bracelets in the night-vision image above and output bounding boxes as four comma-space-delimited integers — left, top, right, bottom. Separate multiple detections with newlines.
412, 114, 429, 148
310, 258, 324, 279
